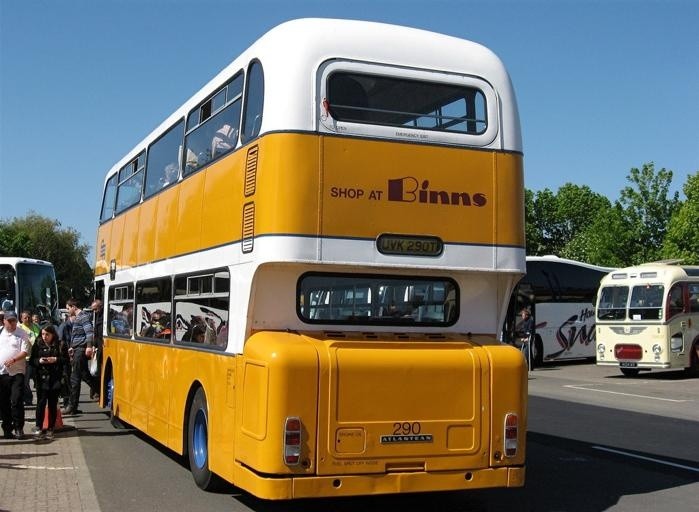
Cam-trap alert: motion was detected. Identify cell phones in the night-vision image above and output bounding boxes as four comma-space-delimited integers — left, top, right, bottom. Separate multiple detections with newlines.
43, 359, 48, 362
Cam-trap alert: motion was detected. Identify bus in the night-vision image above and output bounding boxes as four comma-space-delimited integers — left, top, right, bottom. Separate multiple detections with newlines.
1, 256, 60, 329
90, 18, 530, 501
524, 254, 699, 378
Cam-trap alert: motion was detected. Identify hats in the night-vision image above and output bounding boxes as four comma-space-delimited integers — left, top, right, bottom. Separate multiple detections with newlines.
4, 311, 17, 320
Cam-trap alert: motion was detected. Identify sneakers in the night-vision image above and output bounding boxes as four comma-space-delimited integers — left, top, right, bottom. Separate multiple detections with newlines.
2, 430, 11, 439
34, 429, 43, 439
62, 406, 75, 415
15, 429, 22, 438
46, 429, 54, 440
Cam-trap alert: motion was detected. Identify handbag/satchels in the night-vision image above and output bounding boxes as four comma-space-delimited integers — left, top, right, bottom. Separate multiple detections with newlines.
61, 372, 72, 395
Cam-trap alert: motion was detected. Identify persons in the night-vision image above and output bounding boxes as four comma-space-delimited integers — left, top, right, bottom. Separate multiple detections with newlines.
518, 309, 535, 370
114, 303, 228, 347
0, 298, 116, 441
211, 120, 238, 158
